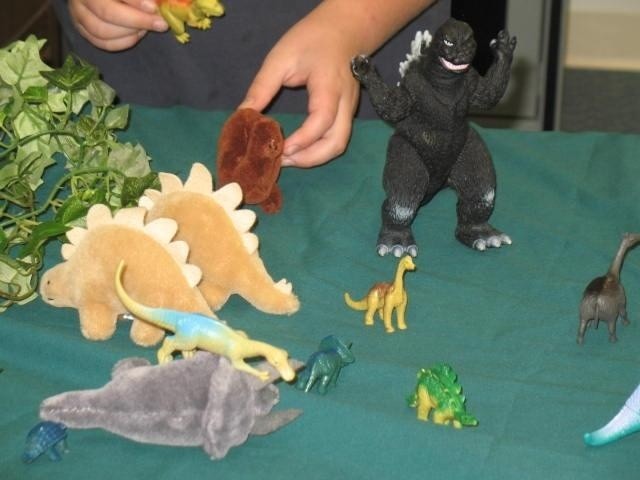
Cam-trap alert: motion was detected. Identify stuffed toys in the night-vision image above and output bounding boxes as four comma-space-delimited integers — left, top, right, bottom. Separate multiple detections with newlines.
216, 108, 284, 212
40, 170, 303, 383
41, 349, 302, 461
20, 420, 70, 464
20, 109, 303, 464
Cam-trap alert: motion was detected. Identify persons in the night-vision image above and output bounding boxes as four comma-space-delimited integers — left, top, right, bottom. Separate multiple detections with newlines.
55, 0, 453, 169
